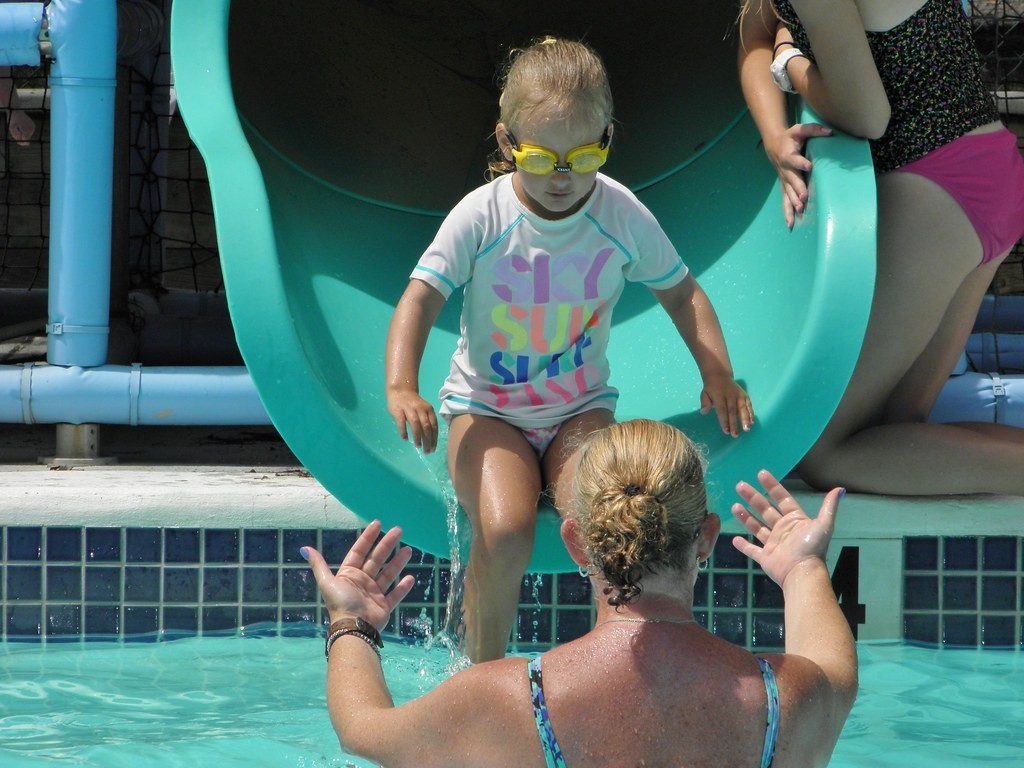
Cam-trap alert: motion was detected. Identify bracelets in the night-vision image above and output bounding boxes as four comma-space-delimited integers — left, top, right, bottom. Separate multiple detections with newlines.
771, 41, 794, 64
324, 616, 384, 660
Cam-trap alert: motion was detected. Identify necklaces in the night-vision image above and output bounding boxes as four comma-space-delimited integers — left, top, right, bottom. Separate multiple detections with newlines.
594, 619, 695, 628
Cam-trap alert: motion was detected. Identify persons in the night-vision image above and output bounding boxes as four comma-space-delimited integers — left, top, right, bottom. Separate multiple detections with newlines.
299, 418, 859, 768
740, 1, 1024, 494
385, 37, 755, 669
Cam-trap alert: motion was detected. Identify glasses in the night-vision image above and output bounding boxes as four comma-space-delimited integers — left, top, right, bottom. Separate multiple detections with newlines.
504, 123, 611, 175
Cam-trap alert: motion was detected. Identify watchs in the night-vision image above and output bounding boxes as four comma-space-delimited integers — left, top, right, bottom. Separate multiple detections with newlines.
769, 47, 807, 95
